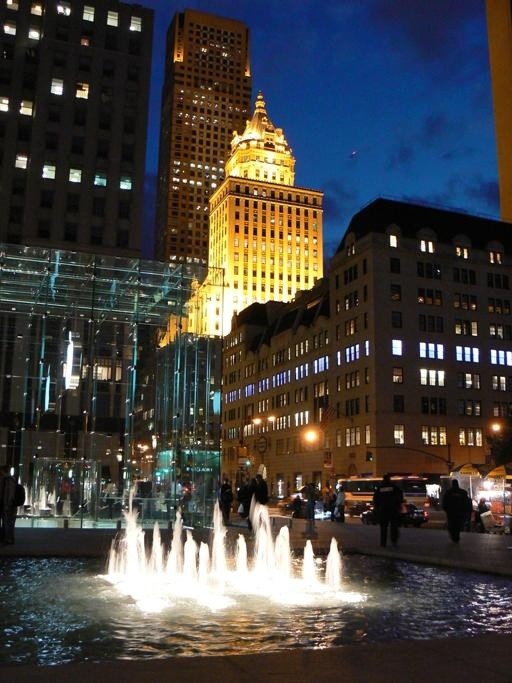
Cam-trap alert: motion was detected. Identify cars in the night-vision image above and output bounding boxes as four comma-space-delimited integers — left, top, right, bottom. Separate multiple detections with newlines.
277, 484, 335, 514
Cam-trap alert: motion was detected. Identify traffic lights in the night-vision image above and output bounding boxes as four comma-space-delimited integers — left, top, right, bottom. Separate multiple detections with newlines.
366, 450, 374, 462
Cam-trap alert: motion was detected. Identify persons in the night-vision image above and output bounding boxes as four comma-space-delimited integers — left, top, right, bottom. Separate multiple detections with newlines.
442, 476, 468, 550
462, 492, 477, 531
371, 469, 406, 548
220, 471, 349, 531
477, 496, 488, 534
1, 463, 21, 550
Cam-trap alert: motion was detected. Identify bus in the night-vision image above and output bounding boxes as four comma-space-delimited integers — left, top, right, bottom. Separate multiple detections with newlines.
334, 475, 430, 527
